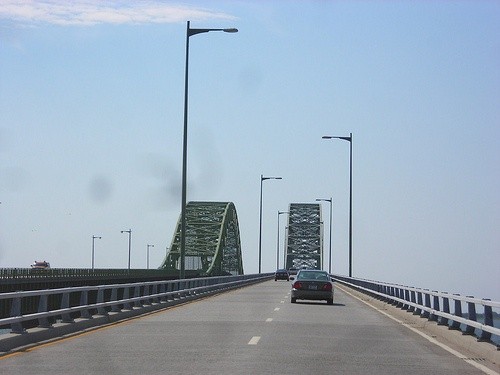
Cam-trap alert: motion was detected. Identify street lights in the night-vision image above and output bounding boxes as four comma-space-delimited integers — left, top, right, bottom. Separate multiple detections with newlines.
120, 228, 132, 270
314, 196, 333, 274
146, 243, 155, 270
276, 209, 290, 271
259, 174, 282, 274
91, 234, 102, 272
321, 132, 353, 277
177, 17, 239, 279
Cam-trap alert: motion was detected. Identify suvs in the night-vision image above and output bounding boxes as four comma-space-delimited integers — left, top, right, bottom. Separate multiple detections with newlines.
30, 260, 51, 275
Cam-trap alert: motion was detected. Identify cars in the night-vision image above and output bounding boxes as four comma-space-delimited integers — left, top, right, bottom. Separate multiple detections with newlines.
273, 269, 291, 282
288, 270, 337, 305
